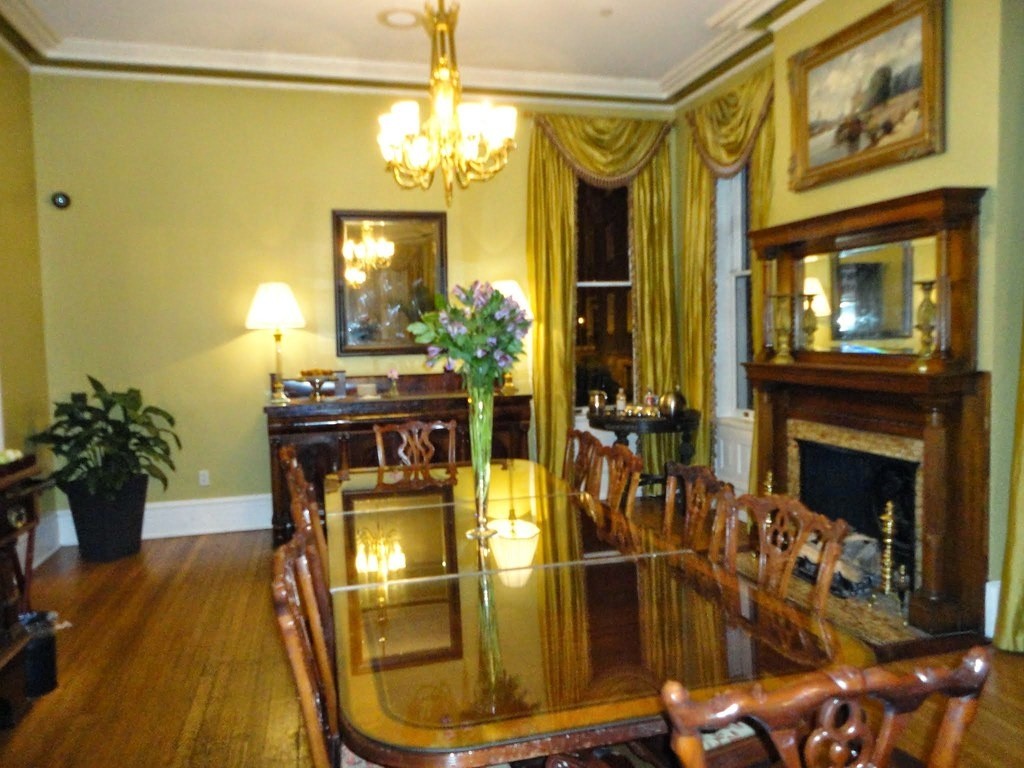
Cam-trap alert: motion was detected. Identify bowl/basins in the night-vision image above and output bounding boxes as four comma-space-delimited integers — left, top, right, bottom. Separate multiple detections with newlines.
357, 382, 377, 398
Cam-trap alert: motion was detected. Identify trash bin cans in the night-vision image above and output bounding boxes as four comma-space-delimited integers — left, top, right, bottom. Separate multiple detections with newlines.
19, 612, 57, 697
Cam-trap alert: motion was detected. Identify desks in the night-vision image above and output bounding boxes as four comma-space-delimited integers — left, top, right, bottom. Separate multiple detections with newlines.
0, 476, 75, 746
322, 457, 880, 768
586, 408, 701, 506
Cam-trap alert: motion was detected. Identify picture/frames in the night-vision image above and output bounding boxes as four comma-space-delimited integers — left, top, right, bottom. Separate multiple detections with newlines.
789, 0, 946, 190
342, 484, 463, 677
831, 238, 914, 339
331, 209, 450, 358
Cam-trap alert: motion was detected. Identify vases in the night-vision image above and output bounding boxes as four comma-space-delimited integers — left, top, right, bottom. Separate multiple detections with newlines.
476, 540, 504, 689
766, 293, 796, 365
908, 279, 942, 373
802, 293, 819, 349
464, 374, 498, 539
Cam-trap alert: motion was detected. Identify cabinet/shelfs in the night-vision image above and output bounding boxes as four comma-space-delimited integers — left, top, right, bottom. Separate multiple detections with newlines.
262, 372, 533, 552
712, 415, 756, 525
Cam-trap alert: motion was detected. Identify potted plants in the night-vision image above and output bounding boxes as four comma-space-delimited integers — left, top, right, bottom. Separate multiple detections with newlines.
22, 373, 183, 563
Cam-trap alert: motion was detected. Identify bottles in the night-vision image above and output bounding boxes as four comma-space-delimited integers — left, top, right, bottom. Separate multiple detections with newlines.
501, 372, 516, 396
588, 384, 685, 418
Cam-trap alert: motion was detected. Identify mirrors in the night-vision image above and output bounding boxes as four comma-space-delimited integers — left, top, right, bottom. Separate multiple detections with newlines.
793, 234, 938, 356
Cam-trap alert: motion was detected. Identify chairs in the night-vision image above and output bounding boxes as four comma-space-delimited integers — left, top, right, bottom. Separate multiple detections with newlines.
662, 461, 737, 565
268, 446, 340, 768
588, 442, 644, 519
722, 488, 852, 608
562, 427, 603, 492
373, 420, 457, 466
545, 645, 993, 768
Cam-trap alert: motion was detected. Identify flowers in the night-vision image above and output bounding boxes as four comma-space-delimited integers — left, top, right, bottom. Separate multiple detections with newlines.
437, 544, 538, 729
387, 368, 400, 382
348, 314, 380, 342
408, 279, 531, 501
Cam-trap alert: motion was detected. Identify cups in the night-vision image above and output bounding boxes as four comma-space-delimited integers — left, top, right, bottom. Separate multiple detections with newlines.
334, 370, 347, 397
487, 520, 540, 588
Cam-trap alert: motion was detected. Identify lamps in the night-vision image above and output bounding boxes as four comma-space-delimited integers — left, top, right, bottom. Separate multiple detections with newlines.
803, 279, 833, 317
376, 0, 517, 208
245, 281, 305, 404
491, 279, 534, 381
343, 221, 395, 289
487, 466, 540, 588
355, 504, 408, 578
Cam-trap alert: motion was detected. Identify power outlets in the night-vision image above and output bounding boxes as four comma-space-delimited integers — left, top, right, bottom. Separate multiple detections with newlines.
199, 469, 210, 487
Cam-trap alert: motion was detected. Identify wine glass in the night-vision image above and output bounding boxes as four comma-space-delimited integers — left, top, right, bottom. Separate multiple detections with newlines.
301, 370, 333, 401
